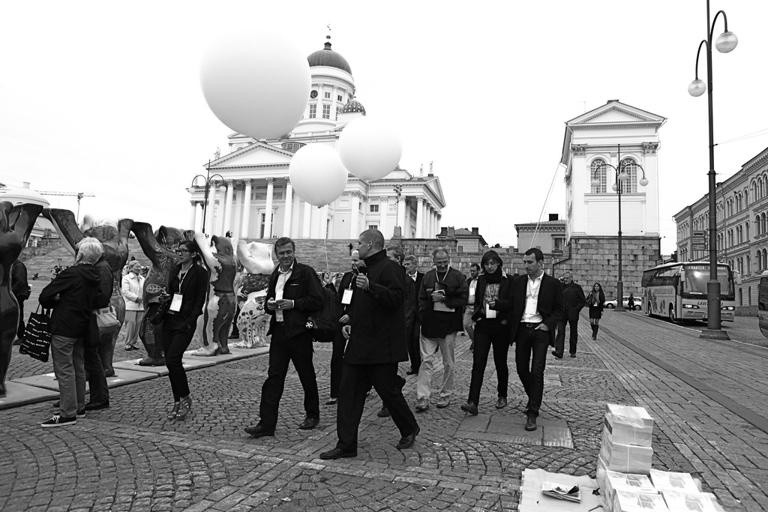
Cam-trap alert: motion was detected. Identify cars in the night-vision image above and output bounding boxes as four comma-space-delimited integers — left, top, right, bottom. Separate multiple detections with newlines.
603, 296, 641, 310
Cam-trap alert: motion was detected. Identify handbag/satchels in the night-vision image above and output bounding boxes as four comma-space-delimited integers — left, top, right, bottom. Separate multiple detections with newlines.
306, 287, 339, 343
96, 307, 121, 337
19, 303, 54, 362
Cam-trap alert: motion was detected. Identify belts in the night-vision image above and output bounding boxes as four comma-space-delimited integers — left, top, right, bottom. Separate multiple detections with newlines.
521, 322, 538, 328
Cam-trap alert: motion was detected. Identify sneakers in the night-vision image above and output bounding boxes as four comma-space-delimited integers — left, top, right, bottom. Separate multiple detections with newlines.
40, 344, 192, 428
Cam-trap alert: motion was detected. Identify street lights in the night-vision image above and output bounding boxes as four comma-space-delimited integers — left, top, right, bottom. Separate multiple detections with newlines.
592, 141, 652, 312
190, 160, 227, 236
390, 182, 403, 237
691, 0, 737, 340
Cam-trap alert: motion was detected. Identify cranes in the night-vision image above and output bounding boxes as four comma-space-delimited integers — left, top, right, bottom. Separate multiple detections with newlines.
35, 191, 96, 225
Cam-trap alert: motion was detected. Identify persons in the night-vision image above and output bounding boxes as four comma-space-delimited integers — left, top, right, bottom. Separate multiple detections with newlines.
585, 283, 604, 340
244, 238, 322, 436
325, 248, 360, 404
38, 241, 102, 428
78, 237, 114, 410
384, 244, 562, 431
551, 271, 585, 358
320, 228, 421, 459
162, 239, 208, 419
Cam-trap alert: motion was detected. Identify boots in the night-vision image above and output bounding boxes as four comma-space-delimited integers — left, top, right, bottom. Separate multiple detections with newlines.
591, 324, 598, 339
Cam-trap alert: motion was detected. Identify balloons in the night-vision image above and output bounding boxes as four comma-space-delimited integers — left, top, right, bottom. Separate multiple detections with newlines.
201, 24, 311, 143
339, 118, 402, 181
289, 142, 347, 208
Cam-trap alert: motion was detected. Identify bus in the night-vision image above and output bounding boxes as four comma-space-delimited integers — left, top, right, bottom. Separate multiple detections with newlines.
757, 271, 768, 337
640, 262, 737, 325
